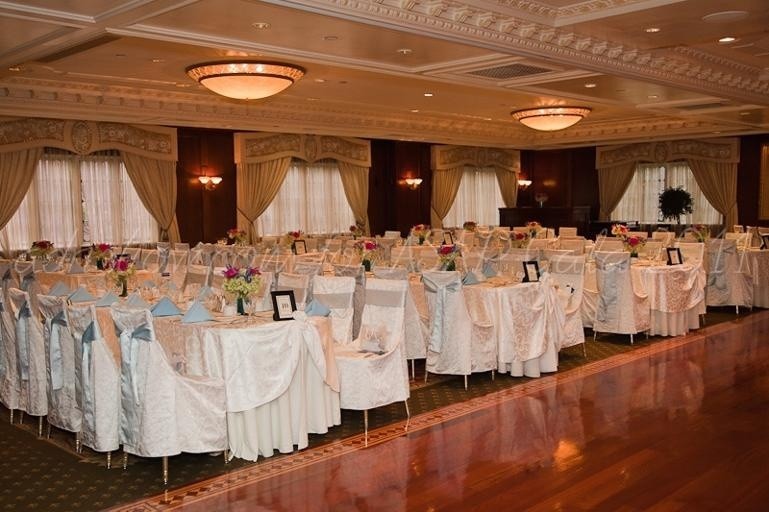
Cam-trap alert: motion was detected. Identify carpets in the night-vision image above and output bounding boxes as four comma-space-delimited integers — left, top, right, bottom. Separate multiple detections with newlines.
0, 305, 769, 512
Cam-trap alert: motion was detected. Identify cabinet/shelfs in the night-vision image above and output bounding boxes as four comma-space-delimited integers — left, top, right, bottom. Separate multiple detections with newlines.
498, 206, 592, 234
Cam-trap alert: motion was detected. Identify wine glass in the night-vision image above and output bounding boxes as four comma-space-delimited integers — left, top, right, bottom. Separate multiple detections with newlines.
85, 274, 263, 326
644, 245, 664, 268
18, 251, 58, 265
212, 237, 231, 247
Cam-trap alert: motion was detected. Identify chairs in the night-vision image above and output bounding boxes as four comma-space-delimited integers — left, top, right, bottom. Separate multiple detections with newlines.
1, 221, 768, 485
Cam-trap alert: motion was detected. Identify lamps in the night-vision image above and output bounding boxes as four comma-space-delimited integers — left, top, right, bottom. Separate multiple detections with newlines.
510, 106, 592, 131
517, 180, 532, 188
198, 176, 223, 190
405, 178, 422, 189
184, 58, 306, 102
